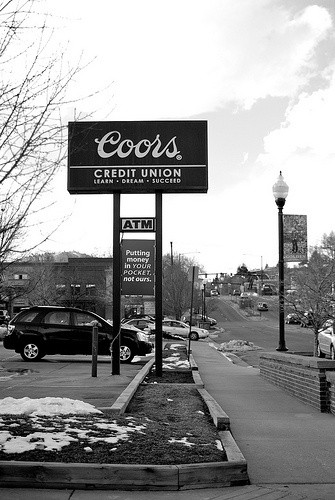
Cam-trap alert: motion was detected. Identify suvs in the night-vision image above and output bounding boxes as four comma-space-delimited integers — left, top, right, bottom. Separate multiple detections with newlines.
3, 305, 153, 364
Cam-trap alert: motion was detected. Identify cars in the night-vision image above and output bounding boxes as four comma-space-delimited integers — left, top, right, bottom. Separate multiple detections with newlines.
285, 313, 301, 325
257, 301, 269, 311
181, 313, 217, 327
232, 288, 249, 299
120, 315, 210, 341
314, 319, 335, 359
209, 289, 219, 296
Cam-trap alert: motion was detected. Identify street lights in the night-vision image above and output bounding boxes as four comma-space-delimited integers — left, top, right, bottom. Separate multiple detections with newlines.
273, 170, 290, 352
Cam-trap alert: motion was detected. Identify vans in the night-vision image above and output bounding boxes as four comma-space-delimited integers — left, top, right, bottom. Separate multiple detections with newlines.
303, 311, 320, 329
